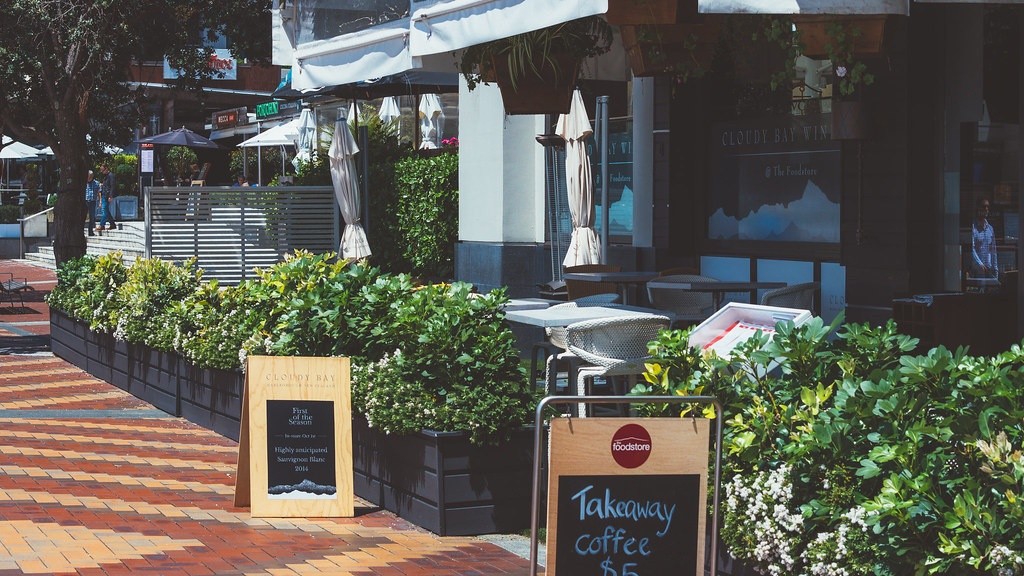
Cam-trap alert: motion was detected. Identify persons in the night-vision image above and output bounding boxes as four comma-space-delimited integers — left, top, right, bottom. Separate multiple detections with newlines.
20, 163, 259, 238
970, 195, 999, 278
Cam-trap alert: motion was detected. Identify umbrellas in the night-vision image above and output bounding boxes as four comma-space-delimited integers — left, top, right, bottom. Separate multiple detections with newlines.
556, 86, 603, 268
327, 106, 371, 264
135, 126, 218, 179
234, 91, 446, 197
0, 134, 124, 189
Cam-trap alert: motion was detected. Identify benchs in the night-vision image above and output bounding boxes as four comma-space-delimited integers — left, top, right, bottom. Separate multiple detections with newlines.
0, 272, 34, 316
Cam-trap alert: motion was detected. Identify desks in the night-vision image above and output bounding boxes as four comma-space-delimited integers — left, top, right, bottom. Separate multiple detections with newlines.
646, 282, 787, 314
504, 308, 669, 418
446, 294, 549, 311
564, 271, 660, 304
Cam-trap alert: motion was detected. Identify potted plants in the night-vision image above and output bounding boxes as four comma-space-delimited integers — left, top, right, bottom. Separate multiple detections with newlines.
621, 6, 721, 78
462, 17, 613, 113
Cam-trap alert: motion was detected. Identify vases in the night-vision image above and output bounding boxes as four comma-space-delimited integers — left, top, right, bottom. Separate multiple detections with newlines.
790, 15, 887, 54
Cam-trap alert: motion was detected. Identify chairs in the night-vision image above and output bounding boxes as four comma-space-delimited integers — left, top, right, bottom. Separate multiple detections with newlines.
543, 274, 819, 417
565, 265, 624, 302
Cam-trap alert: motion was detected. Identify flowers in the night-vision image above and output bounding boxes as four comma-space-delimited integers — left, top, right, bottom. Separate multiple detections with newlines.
750, 15, 876, 93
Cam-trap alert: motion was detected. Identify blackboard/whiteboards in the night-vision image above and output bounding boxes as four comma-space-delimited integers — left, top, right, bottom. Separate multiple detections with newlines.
185, 180, 211, 216
232, 355, 354, 518
545, 419, 712, 576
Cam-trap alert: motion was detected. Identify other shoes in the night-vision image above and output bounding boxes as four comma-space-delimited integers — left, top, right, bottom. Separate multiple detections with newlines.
108, 224, 116, 230
96, 225, 105, 230
89, 231, 94, 235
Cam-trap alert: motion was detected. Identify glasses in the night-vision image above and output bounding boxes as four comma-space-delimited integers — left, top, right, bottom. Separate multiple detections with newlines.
981, 205, 990, 209
